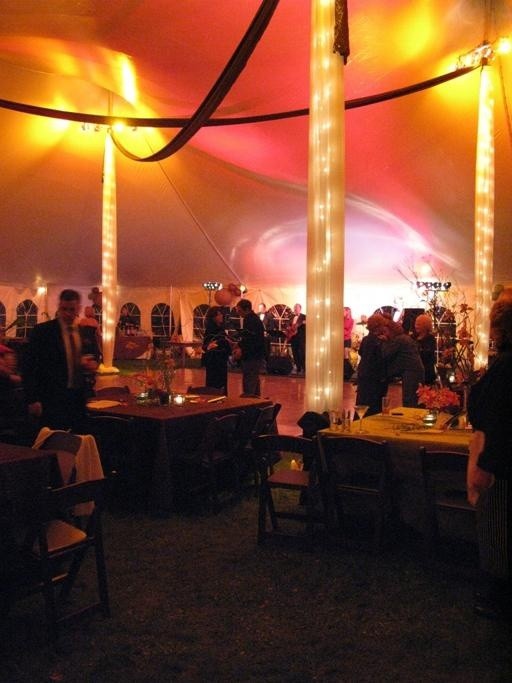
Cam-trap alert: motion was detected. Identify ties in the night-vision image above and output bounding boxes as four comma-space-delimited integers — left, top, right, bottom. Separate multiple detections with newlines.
65, 326, 79, 376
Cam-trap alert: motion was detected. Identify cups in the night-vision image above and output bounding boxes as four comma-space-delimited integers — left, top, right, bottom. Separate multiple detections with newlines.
327, 407, 340, 431
79, 353, 96, 375
381, 396, 391, 416
341, 407, 351, 432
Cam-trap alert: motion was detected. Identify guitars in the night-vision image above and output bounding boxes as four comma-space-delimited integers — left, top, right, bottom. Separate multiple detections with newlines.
286, 322, 303, 342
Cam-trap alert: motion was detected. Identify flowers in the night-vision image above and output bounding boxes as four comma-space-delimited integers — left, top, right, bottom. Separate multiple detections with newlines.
156, 348, 177, 382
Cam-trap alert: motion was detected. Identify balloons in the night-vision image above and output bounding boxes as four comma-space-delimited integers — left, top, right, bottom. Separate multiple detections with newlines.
492, 291, 499, 301
215, 283, 241, 305
494, 284, 504, 294
88, 286, 102, 314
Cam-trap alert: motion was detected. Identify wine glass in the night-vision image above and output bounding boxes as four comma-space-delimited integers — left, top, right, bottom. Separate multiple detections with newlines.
352, 405, 370, 434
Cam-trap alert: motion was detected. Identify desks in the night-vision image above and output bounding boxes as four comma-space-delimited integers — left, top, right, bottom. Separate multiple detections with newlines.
160, 340, 203, 377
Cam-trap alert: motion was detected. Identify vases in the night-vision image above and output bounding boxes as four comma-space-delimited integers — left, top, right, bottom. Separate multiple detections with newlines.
159, 366, 175, 396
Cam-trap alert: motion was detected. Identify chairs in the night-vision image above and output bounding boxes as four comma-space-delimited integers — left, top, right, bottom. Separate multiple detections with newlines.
0, 424, 110, 682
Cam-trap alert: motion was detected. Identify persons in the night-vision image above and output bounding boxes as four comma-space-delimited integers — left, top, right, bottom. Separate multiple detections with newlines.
201, 297, 439, 423
78, 305, 104, 364
15, 289, 102, 447
117, 305, 136, 329
463, 289, 512, 620
0, 344, 41, 446
